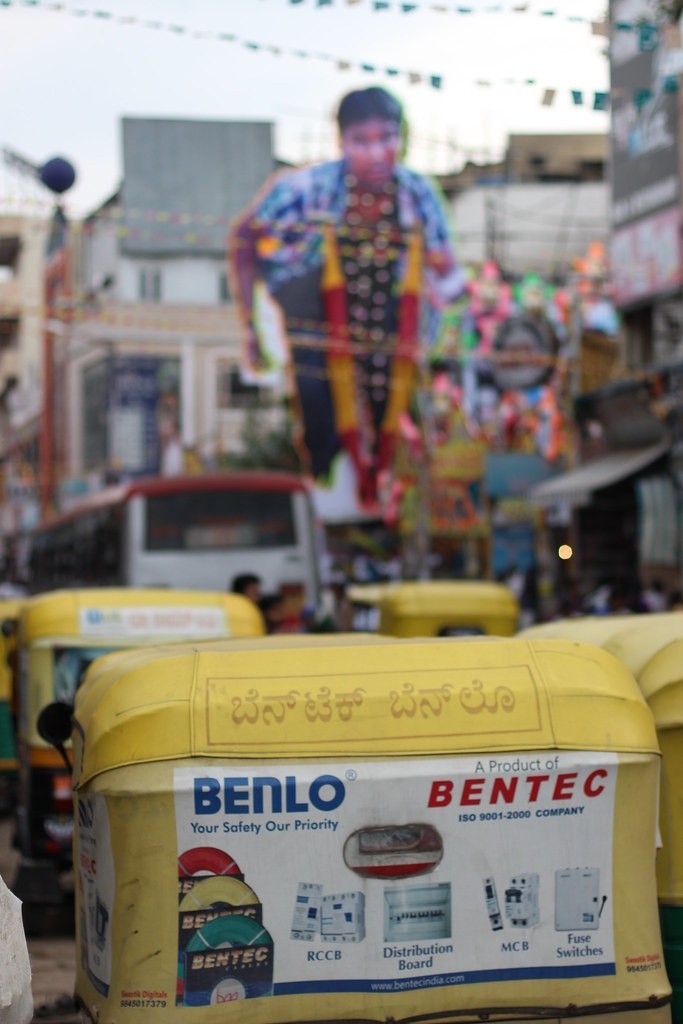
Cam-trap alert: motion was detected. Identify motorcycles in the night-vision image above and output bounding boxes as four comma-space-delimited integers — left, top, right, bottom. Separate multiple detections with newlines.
34, 630, 675, 1024
511, 611, 681, 907
0, 589, 270, 899
342, 580, 519, 638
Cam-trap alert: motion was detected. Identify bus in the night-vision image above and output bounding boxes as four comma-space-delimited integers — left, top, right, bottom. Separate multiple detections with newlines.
5, 470, 334, 635
